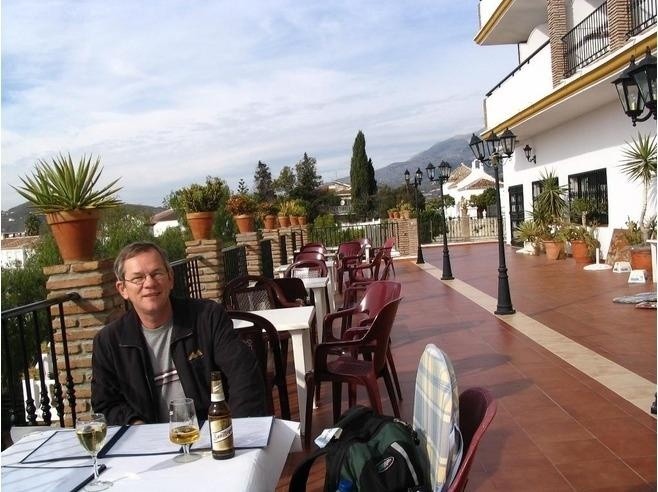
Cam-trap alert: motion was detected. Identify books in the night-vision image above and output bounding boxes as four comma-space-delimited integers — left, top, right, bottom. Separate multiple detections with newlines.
190, 415, 274, 454
21, 424, 185, 459
2, 465, 107, 492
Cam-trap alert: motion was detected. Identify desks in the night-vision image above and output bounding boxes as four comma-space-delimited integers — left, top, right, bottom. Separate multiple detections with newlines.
302, 278, 330, 345
288, 253, 336, 264
294, 246, 338, 253
230, 306, 318, 437
275, 261, 338, 312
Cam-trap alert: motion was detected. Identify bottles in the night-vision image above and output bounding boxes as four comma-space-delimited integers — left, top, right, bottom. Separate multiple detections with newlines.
208, 372, 235, 460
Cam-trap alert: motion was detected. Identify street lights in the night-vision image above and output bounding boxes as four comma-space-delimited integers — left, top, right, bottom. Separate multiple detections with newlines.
470, 128, 524, 315
612, 47, 657, 127
404, 167, 424, 266
427, 161, 452, 280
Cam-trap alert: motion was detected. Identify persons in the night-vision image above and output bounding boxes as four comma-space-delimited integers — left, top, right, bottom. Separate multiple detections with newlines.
91, 243, 268, 426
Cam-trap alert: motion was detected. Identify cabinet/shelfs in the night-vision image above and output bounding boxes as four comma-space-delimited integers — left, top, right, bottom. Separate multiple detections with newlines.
341, 257, 392, 338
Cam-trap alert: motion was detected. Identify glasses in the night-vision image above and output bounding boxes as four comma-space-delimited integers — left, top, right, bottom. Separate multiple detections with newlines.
121, 270, 170, 286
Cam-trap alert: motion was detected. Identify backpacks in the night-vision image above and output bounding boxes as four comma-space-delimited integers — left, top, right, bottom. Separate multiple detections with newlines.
286, 405, 434, 492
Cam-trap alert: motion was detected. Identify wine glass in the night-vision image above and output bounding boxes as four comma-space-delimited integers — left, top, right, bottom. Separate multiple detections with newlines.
76, 413, 113, 492
168, 398, 202, 463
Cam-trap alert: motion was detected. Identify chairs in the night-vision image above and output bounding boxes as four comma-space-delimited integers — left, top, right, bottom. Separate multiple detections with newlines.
335, 242, 361, 282
293, 252, 327, 261
224, 275, 299, 374
229, 311, 291, 420
255, 278, 317, 347
322, 281, 403, 409
305, 296, 403, 446
348, 247, 391, 281
307, 242, 323, 245
301, 245, 326, 252
370, 238, 396, 279
444, 387, 497, 492
285, 261, 327, 278
352, 238, 368, 255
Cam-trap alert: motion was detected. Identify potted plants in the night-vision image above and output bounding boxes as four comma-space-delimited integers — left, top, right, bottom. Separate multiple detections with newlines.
515, 221, 540, 256
224, 194, 259, 233
7, 153, 126, 263
254, 202, 281, 230
525, 168, 565, 260
614, 131, 657, 277
560, 198, 600, 265
272, 203, 290, 229
390, 207, 396, 217
287, 202, 298, 226
177, 181, 224, 240
299, 206, 308, 224
385, 209, 390, 216
403, 203, 411, 218
397, 204, 402, 216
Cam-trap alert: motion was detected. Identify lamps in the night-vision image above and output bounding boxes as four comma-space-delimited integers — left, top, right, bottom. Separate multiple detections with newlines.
524, 145, 536, 164
